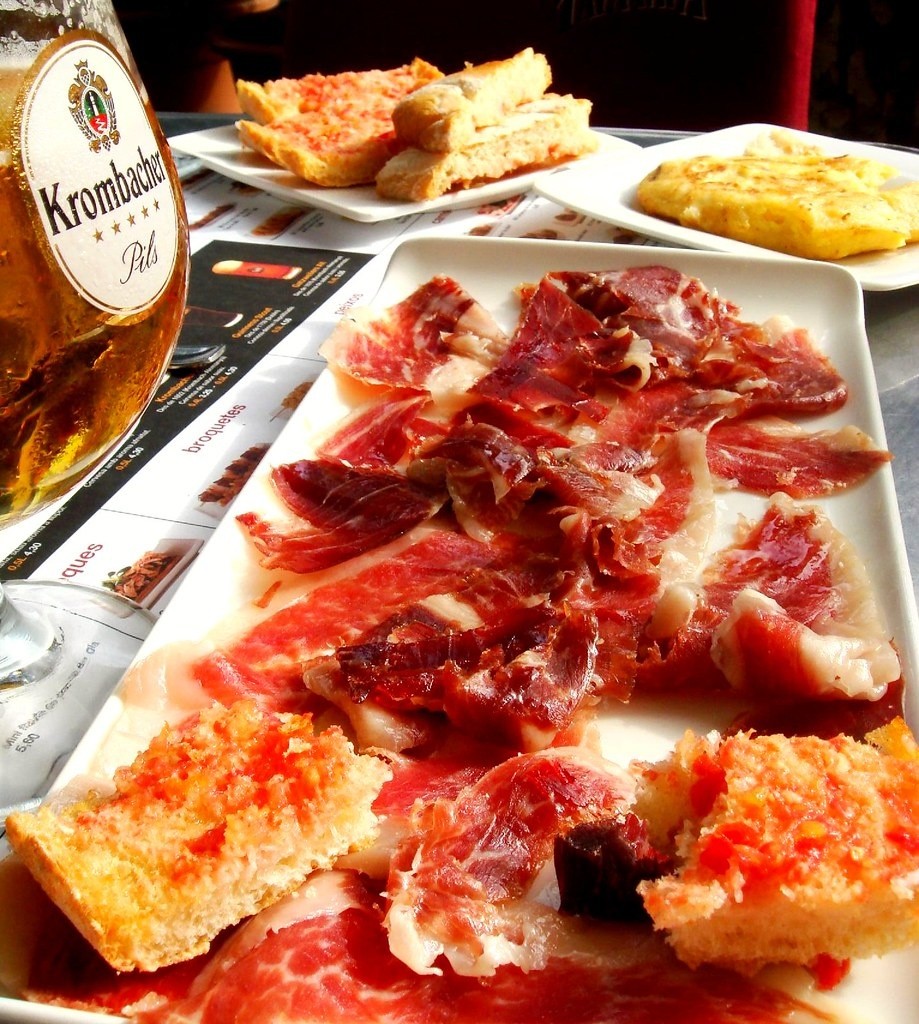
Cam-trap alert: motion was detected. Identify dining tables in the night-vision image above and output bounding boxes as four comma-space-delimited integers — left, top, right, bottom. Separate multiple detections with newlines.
0, 110, 919, 1024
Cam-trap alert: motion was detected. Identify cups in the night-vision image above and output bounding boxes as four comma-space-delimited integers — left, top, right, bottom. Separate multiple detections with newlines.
182, 306, 243, 328
212, 259, 301, 280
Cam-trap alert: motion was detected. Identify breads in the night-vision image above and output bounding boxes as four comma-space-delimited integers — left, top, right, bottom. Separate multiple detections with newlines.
5, 700, 394, 969
234, 48, 594, 206
630, 713, 918, 965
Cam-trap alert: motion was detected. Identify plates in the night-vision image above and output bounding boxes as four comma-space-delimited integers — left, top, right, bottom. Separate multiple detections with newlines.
194, 441, 273, 520
246, 204, 320, 241
92, 538, 205, 618
0, 236, 919, 1024
270, 373, 319, 420
531, 123, 919, 291
186, 201, 238, 230
166, 122, 643, 224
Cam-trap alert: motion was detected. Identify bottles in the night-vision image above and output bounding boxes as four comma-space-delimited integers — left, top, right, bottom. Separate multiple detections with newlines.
0, 0, 190, 533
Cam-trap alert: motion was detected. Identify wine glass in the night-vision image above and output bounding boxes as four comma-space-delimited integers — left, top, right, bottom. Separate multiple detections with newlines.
0, 580, 158, 828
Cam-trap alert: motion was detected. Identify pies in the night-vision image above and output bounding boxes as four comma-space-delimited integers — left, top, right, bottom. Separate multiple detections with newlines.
632, 147, 919, 261
